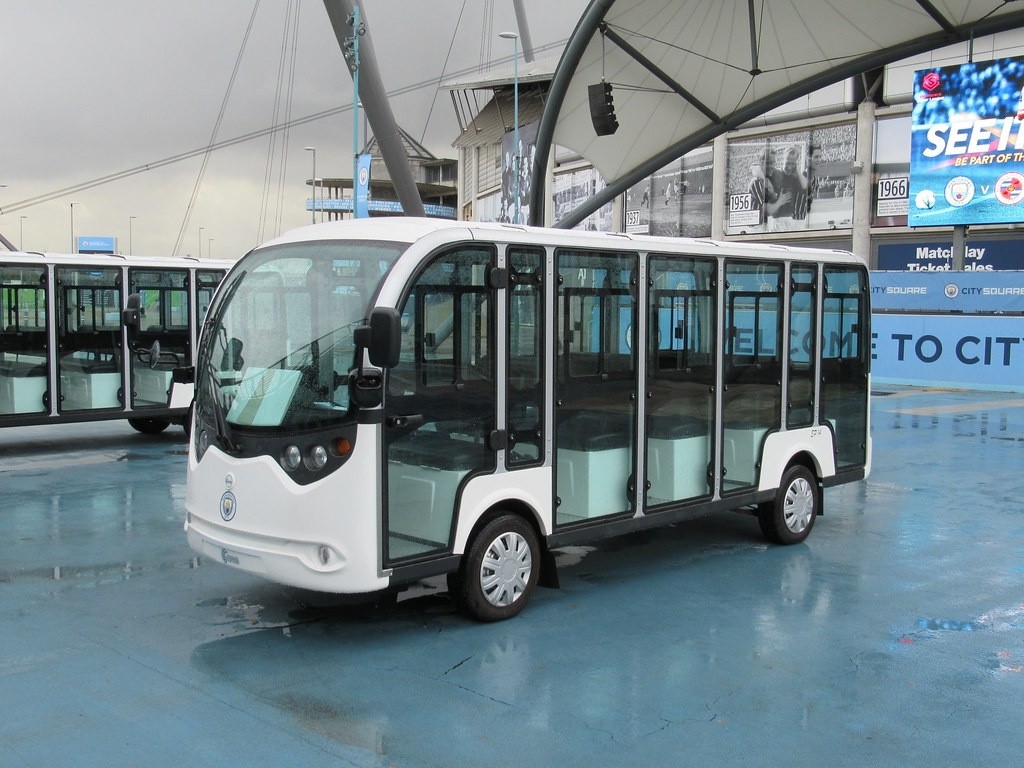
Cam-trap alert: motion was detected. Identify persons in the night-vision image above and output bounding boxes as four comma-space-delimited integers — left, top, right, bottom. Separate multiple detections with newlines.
640, 179, 705, 209
793, 145, 823, 220
751, 147, 809, 218
500, 136, 538, 223
747, 147, 792, 224
816, 175, 854, 200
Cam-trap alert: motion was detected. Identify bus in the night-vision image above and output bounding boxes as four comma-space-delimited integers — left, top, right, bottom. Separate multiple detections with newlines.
184, 217, 876, 622
0, 251, 239, 437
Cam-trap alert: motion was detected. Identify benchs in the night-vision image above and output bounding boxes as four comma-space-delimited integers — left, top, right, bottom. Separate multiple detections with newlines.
2, 323, 199, 368
371, 363, 827, 472
